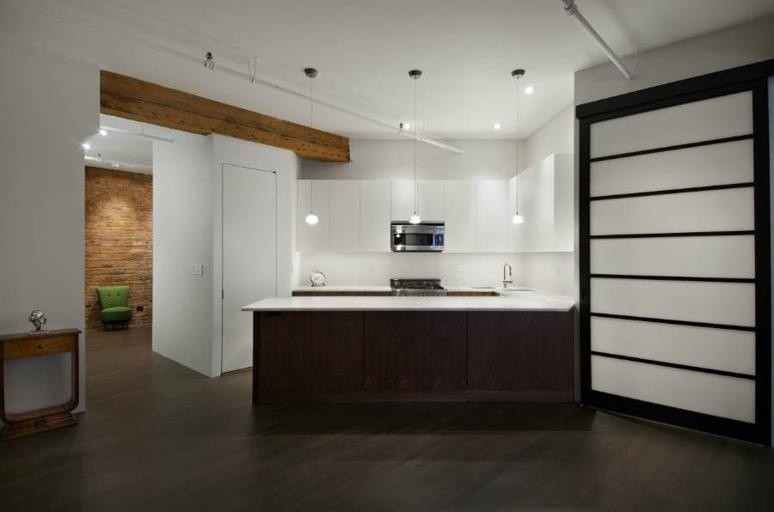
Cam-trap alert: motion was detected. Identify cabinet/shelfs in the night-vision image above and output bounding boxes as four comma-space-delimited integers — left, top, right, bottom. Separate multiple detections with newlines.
300, 179, 390, 254
444, 179, 508, 254
0, 328, 83, 443
508, 153, 575, 254
387, 182, 444, 220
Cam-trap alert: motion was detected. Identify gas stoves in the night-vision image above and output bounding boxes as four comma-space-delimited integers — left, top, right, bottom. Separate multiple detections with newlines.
389, 276, 448, 292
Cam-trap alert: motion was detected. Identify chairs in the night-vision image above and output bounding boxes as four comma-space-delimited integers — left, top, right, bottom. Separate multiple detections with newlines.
94, 283, 135, 330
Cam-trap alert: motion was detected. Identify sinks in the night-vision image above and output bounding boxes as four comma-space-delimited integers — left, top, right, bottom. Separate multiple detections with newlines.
502, 289, 534, 291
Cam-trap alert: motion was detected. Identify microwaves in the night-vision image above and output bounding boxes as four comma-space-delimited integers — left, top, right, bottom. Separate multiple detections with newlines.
391, 222, 445, 251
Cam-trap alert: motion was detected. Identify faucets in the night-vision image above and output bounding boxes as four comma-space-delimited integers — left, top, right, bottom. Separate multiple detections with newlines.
502, 261, 512, 288
